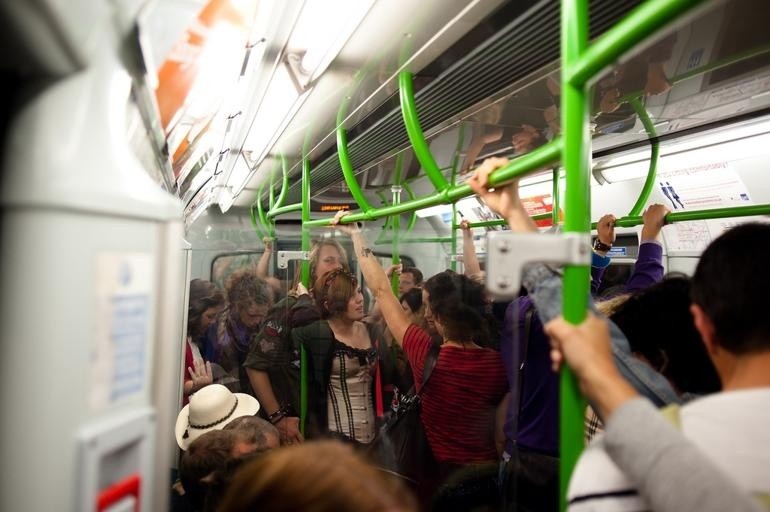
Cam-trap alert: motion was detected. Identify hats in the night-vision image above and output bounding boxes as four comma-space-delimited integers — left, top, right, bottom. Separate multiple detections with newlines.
174, 385, 261, 451
205, 362, 240, 385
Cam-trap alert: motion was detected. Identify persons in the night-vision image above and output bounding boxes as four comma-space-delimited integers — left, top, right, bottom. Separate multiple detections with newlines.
461, 42, 674, 176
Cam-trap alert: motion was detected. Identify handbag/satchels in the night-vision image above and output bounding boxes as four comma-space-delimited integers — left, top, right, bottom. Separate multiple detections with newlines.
367, 388, 423, 469
